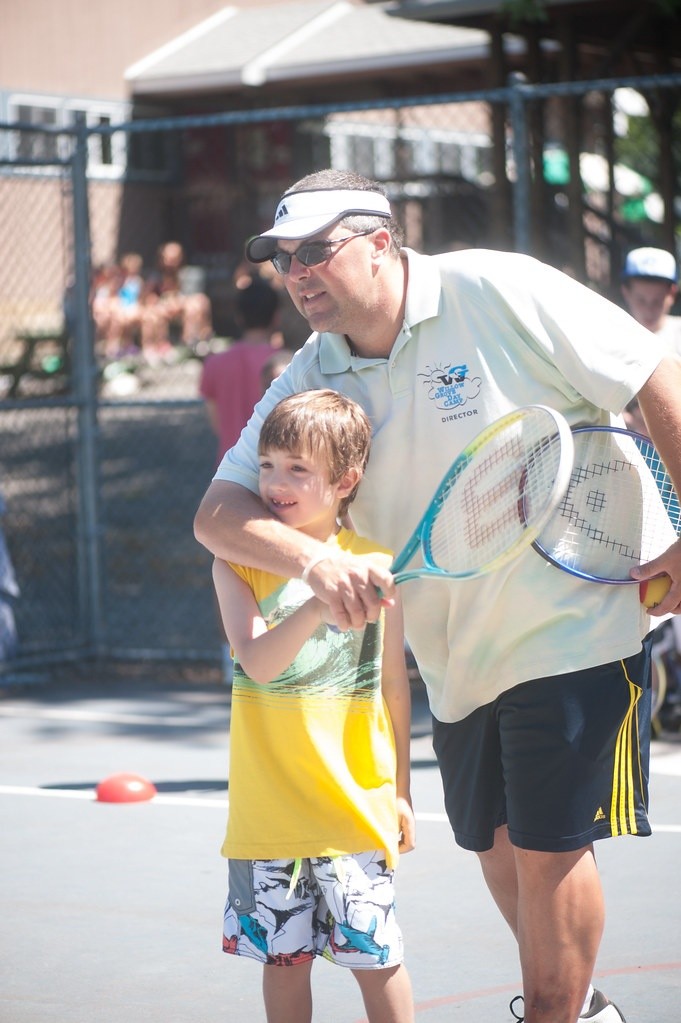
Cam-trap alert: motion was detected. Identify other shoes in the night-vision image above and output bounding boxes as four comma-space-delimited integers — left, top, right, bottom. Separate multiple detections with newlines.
509, 988, 626, 1023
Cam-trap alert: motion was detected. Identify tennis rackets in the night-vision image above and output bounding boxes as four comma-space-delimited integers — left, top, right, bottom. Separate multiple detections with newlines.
325, 402, 575, 636
519, 424, 681, 585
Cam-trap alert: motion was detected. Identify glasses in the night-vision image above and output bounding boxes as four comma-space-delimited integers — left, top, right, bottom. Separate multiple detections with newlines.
269, 227, 400, 275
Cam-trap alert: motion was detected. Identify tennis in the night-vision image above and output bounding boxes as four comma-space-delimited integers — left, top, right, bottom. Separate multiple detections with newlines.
639, 573, 673, 609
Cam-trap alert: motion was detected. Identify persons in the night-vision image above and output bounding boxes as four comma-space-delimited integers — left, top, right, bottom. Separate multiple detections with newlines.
199, 282, 299, 467
195, 168, 681, 1023
211, 390, 417, 1023
611, 246, 681, 440
72, 240, 214, 396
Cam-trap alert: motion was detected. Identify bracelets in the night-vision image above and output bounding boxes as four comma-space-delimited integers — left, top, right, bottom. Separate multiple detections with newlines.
302, 553, 334, 585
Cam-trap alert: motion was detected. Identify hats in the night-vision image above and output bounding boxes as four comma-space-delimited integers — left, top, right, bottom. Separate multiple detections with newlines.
244, 188, 393, 264
623, 246, 678, 283
